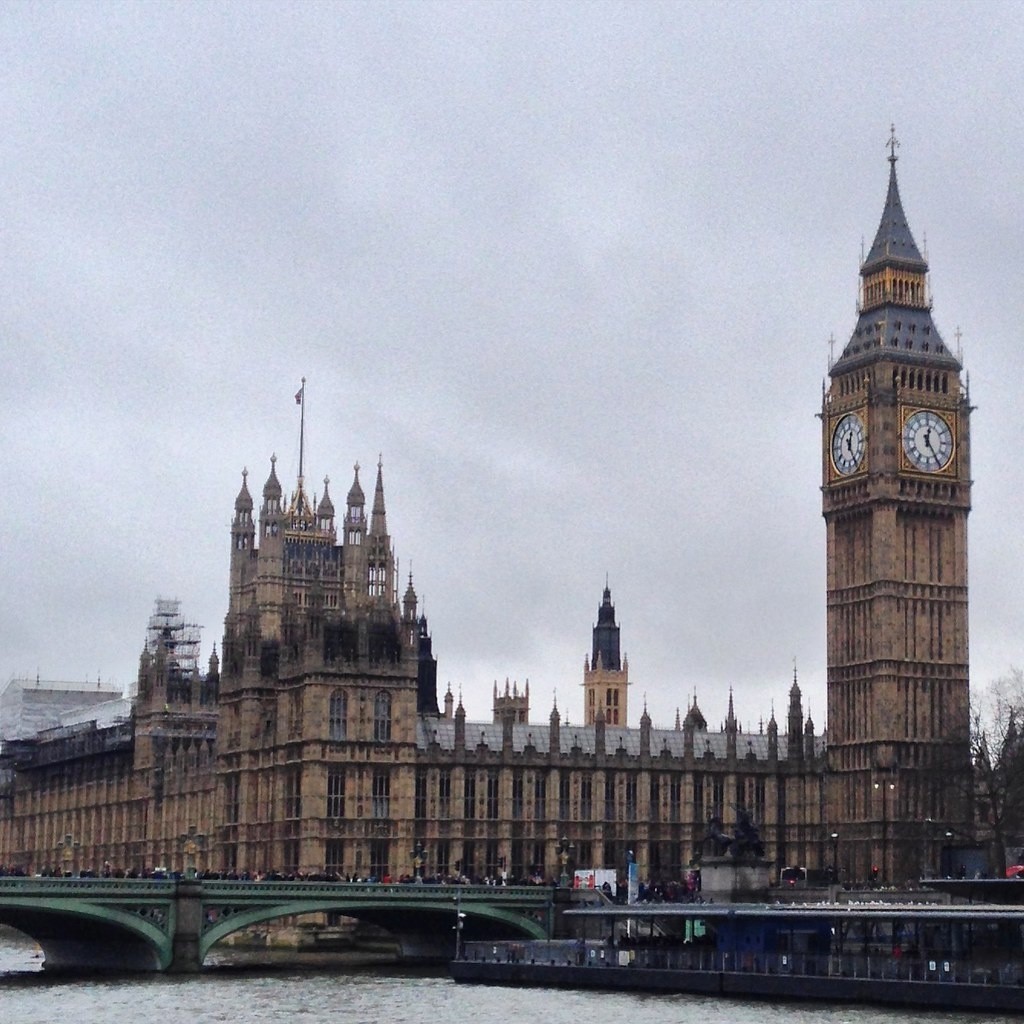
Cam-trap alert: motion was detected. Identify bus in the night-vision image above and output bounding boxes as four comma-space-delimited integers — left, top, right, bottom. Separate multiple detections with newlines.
780, 868, 807, 889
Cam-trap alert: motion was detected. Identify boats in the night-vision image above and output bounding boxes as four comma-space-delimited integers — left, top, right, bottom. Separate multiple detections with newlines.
448, 893, 1023, 1011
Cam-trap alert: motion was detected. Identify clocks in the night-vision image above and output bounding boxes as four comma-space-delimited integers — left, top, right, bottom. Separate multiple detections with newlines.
829, 406, 869, 479
899, 404, 956, 477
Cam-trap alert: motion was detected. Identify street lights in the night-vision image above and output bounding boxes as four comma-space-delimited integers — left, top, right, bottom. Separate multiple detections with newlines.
56, 834, 80, 878
831, 830, 839, 884
416, 841, 421, 877
946, 831, 954, 880
560, 835, 569, 887
180, 824, 203, 878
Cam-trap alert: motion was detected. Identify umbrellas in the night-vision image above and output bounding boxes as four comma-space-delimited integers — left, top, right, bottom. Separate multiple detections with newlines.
1005, 864, 1024, 878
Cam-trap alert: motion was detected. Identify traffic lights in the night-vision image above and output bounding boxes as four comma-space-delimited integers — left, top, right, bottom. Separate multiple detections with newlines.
872, 866, 878, 881
688, 872, 695, 891
455, 860, 459, 871
497, 857, 502, 868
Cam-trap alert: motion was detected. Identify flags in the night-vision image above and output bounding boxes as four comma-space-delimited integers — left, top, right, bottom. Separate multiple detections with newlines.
295, 387, 304, 404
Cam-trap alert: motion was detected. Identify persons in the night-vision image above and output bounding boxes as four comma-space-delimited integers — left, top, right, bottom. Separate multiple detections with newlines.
384, 873, 394, 894
958, 865, 966, 880
400, 869, 469, 884
352, 872, 359, 883
287, 870, 339, 882
346, 872, 350, 882
0, 860, 268, 881
274, 870, 283, 881
481, 866, 549, 887
639, 875, 688, 903
